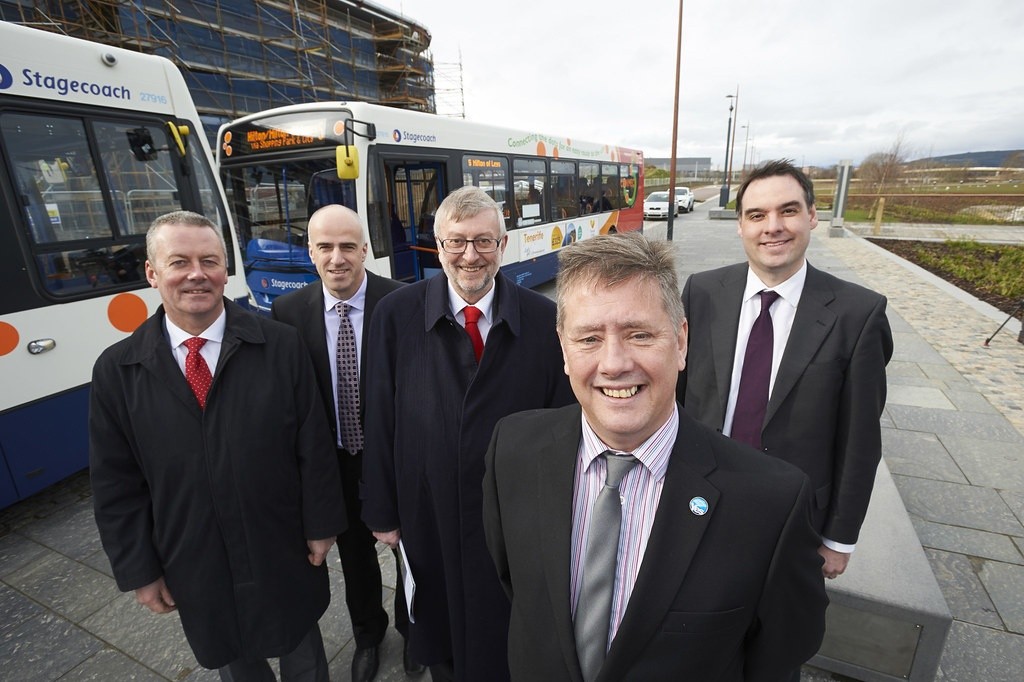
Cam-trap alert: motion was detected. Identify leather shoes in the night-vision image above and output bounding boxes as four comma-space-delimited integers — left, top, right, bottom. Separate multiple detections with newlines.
351, 629, 387, 682
403, 636, 425, 675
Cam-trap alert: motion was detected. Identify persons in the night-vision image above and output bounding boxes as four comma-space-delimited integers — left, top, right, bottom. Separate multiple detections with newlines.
271, 203, 429, 682
483, 230, 831, 682
676, 158, 894, 682
503, 175, 637, 227
89, 210, 350, 682
361, 185, 579, 682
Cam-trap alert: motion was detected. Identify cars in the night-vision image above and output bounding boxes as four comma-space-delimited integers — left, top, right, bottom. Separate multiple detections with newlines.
478, 186, 506, 210
667, 187, 695, 213
643, 191, 678, 219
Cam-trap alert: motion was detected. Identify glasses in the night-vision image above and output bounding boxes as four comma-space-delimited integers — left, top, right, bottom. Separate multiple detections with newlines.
438, 235, 502, 254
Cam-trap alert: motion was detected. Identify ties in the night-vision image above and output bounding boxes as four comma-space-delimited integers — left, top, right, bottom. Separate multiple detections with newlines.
462, 306, 485, 364
335, 301, 365, 457
572, 452, 641, 681
728, 291, 781, 451
181, 337, 214, 413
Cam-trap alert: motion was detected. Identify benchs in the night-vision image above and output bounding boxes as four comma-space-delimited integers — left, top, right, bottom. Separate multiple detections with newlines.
806, 456, 954, 682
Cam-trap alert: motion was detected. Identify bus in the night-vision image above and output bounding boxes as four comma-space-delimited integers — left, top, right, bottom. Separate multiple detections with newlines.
212, 99, 645, 313
0, 23, 256, 508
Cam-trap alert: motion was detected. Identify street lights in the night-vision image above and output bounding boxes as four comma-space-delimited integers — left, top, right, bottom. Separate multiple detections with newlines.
720, 95, 733, 205
741, 125, 749, 180
